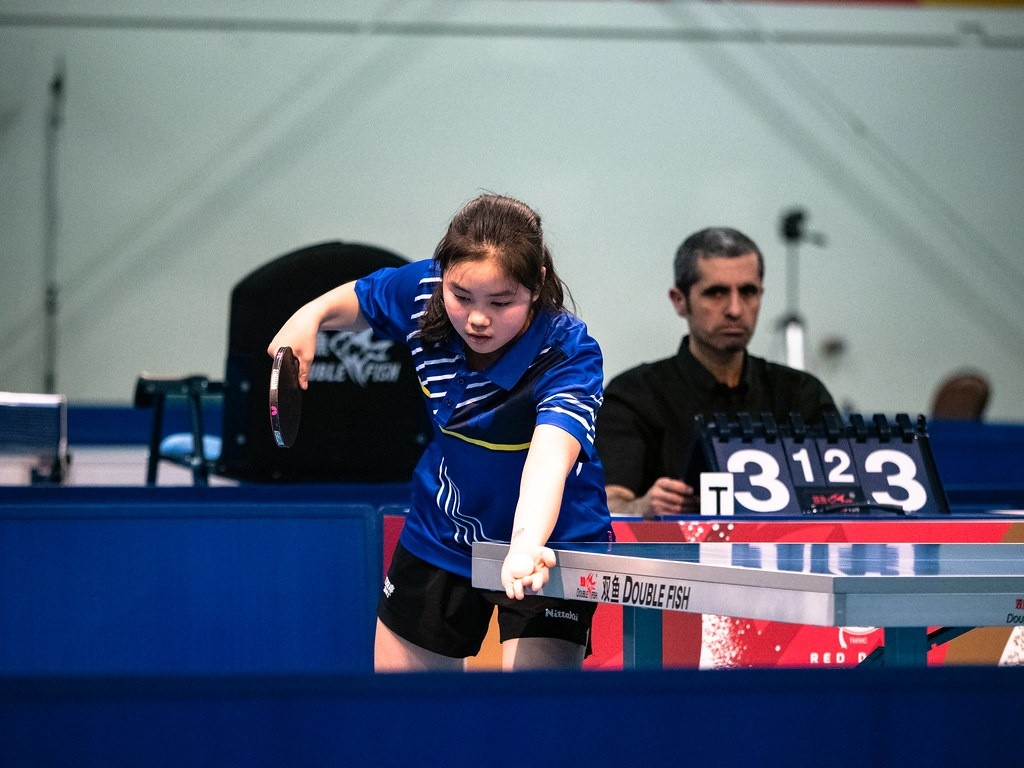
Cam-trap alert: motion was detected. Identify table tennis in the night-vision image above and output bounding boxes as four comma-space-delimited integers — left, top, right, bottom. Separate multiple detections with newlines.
507, 550, 535, 583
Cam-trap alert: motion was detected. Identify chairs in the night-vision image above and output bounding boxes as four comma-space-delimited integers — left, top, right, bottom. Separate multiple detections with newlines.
131, 240, 435, 486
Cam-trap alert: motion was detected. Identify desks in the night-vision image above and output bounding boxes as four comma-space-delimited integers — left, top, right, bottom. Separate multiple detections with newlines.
468, 541, 1024, 667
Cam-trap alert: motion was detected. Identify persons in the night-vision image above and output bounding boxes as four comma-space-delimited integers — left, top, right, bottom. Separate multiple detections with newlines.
592, 227, 849, 521
264, 196, 615, 670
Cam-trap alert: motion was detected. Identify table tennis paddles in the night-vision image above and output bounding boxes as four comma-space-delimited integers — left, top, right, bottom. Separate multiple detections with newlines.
269, 347, 305, 449
